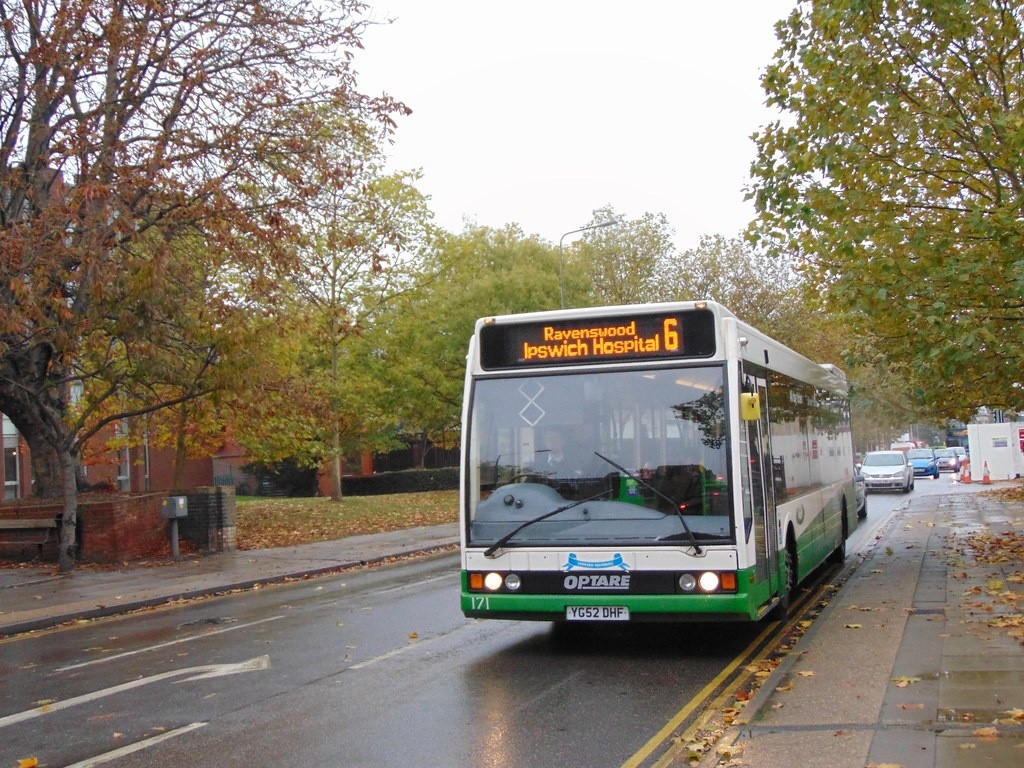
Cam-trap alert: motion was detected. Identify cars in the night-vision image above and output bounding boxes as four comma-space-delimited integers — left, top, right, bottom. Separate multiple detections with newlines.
907, 448, 940, 479
934, 448, 960, 473
853, 450, 913, 518
945, 445, 970, 466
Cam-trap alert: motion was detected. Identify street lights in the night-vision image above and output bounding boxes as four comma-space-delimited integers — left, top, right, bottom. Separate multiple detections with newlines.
560, 220, 619, 310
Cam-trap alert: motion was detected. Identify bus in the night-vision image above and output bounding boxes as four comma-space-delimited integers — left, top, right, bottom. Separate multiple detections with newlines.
458, 298, 858, 622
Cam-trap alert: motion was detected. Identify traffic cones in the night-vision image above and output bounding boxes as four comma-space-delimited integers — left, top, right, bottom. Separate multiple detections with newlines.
978, 460, 994, 484
957, 459, 974, 484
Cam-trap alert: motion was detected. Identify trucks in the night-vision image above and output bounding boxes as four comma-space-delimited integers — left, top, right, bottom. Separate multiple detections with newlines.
890, 441, 920, 452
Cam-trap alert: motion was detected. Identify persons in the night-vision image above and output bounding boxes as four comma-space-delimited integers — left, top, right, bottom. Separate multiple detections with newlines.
522, 429, 587, 502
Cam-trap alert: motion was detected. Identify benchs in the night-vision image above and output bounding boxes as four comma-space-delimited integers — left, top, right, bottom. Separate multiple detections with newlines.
0, 511, 62, 562
